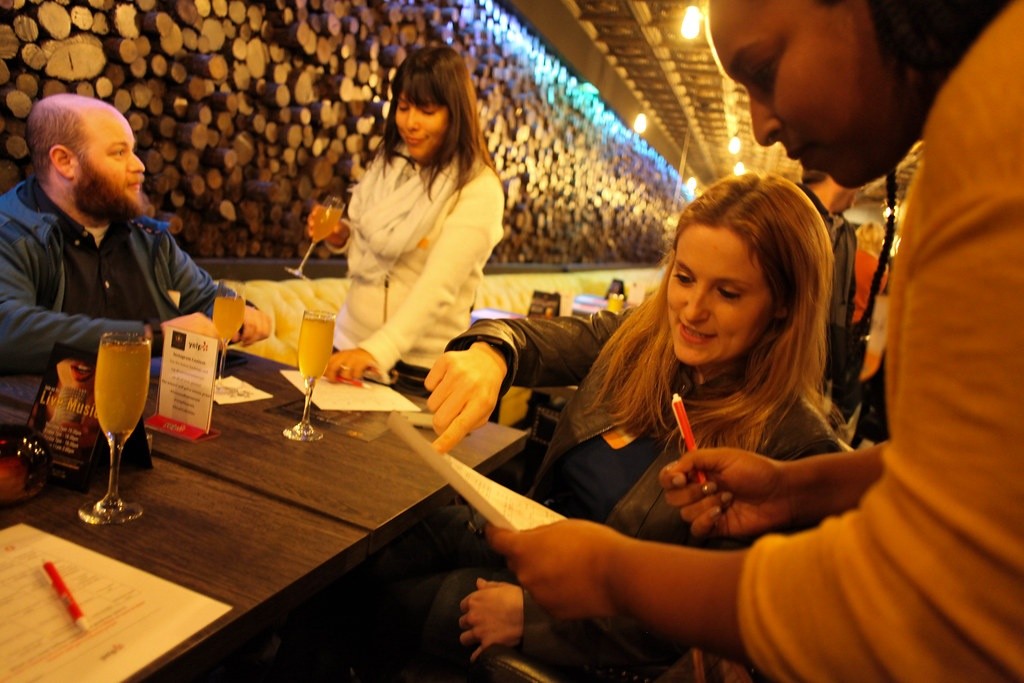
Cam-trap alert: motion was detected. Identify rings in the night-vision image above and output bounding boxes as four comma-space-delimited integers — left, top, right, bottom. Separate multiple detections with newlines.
341, 365, 350, 369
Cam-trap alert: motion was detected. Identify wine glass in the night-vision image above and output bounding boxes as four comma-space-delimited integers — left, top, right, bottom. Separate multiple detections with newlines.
282, 311, 335, 441
212, 279, 248, 397
78, 333, 152, 525
285, 195, 346, 282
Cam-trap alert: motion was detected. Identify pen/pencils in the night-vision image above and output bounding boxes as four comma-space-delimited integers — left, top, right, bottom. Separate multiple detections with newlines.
671, 393, 708, 492
323, 373, 372, 389
39, 557, 90, 633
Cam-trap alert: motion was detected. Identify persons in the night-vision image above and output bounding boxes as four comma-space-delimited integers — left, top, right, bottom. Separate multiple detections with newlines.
484, 0, 1024, 683
1, 94, 272, 435
272, 174, 832, 683
306, 49, 506, 397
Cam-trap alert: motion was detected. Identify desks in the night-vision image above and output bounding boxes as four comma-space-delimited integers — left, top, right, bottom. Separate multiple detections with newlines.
0, 347, 532, 683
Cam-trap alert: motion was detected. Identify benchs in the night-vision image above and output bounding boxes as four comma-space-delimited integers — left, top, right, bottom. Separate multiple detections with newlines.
166, 258, 665, 368
